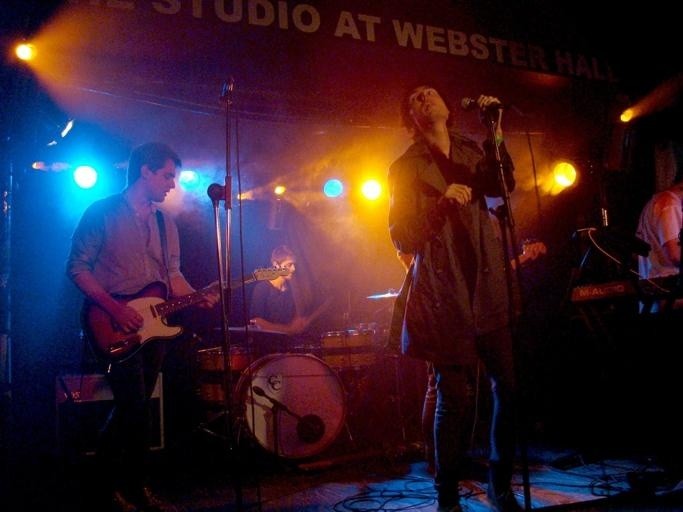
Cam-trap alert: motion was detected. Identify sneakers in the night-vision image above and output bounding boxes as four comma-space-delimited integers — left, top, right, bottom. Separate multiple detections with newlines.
434, 502, 463, 512
489, 497, 521, 511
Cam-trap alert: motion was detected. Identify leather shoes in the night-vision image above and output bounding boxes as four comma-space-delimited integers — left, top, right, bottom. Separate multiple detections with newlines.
106, 488, 137, 512
138, 486, 178, 512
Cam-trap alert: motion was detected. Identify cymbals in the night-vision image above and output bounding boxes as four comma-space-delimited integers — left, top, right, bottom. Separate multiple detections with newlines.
367, 294, 398, 299
214, 324, 286, 336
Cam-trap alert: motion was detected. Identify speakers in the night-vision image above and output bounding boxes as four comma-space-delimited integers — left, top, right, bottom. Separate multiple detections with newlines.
53, 363, 167, 454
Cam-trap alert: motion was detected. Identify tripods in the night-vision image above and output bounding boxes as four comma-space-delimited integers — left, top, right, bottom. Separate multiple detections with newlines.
192, 103, 302, 512
250, 387, 306, 486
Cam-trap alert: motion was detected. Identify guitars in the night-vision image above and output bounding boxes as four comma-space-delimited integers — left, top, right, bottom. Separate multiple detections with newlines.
80, 265, 289, 364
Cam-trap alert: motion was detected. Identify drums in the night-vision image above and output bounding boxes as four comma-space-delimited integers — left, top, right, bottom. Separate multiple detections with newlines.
197, 344, 259, 407
232, 352, 348, 460
321, 329, 389, 371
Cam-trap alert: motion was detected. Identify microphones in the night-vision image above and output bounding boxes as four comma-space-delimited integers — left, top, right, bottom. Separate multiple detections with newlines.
600, 197, 609, 228
220, 73, 234, 100
304, 419, 321, 435
461, 97, 511, 112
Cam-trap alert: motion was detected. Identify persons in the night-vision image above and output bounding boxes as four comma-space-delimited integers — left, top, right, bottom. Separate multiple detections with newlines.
391, 83, 527, 512
634, 170, 683, 296
67, 140, 219, 510
249, 244, 305, 334
